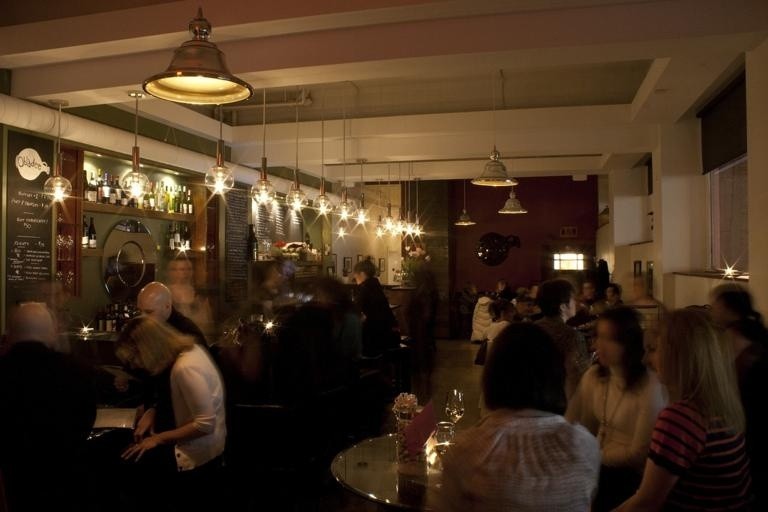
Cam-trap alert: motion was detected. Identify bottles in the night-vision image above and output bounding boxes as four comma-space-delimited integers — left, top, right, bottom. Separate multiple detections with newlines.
166, 222, 193, 252
83, 166, 195, 217
395, 473, 425, 510
248, 223, 258, 260
82, 214, 96, 248
93, 302, 135, 335
395, 410, 429, 477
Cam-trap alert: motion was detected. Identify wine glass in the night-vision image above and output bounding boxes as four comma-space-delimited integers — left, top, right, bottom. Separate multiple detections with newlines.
434, 422, 455, 469
444, 387, 466, 422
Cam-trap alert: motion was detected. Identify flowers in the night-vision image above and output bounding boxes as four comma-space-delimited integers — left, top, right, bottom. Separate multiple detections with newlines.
396, 246, 431, 278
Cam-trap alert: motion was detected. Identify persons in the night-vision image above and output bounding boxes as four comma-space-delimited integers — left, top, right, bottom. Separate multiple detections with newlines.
614, 308, 754, 511
34, 277, 91, 347
406, 272, 442, 401
710, 283, 767, 371
440, 323, 601, 510
1, 302, 97, 511
165, 250, 403, 403
569, 306, 668, 510
471, 252, 623, 358
112, 315, 228, 508
119, 283, 207, 430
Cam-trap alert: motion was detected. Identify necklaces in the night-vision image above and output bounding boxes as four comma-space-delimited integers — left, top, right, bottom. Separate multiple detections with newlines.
601, 373, 629, 442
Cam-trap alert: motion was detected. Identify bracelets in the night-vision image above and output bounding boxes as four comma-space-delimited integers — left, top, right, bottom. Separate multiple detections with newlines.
158, 431, 170, 444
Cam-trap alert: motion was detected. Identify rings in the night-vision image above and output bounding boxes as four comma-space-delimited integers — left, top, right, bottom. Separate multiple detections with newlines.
135, 443, 138, 447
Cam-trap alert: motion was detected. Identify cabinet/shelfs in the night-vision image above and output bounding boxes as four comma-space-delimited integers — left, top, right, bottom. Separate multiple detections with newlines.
79, 148, 207, 262
607, 167, 649, 249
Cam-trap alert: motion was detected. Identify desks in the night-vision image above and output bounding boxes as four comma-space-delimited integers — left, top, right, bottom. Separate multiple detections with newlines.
325, 426, 479, 512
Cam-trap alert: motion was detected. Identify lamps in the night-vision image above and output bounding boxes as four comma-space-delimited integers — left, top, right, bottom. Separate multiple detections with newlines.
334, 107, 355, 222
497, 185, 528, 214
312, 88, 333, 215
470, 143, 518, 187
251, 84, 277, 208
202, 103, 237, 197
453, 179, 477, 226
42, 96, 74, 202
373, 199, 428, 240
354, 156, 371, 224
123, 87, 150, 200
142, 0, 257, 113
284, 84, 309, 212
551, 245, 585, 272
